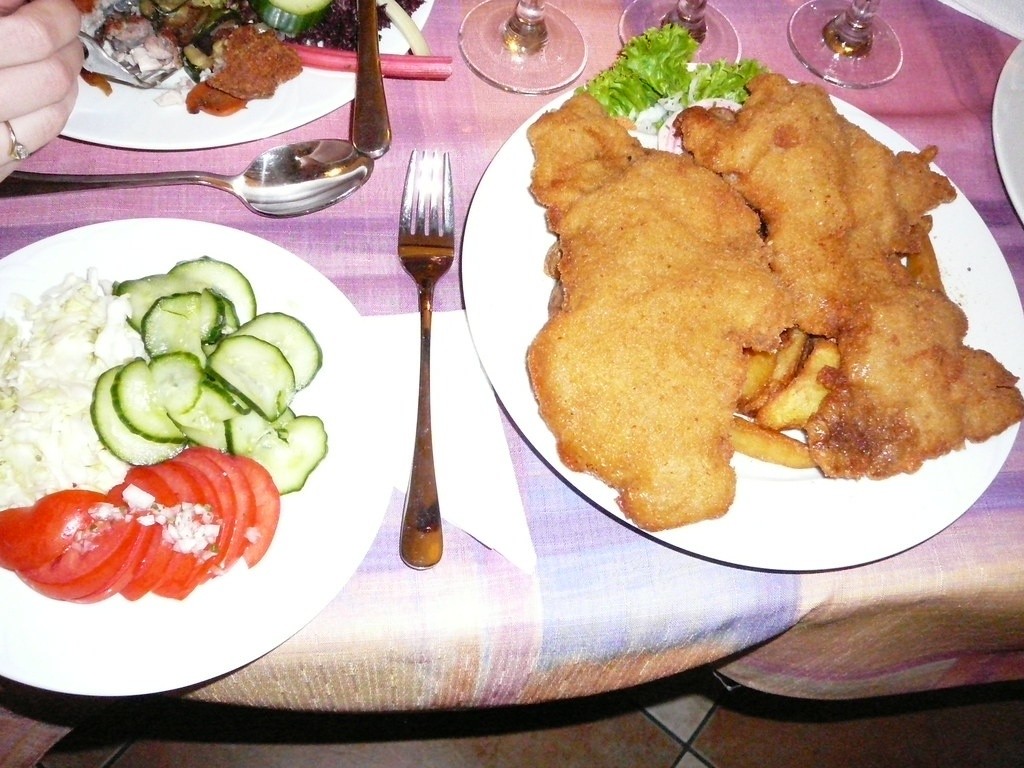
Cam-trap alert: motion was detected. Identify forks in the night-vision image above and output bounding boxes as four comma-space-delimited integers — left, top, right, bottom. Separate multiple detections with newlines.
396, 149, 454, 571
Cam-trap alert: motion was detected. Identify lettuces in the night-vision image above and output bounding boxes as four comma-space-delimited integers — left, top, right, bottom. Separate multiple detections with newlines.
575, 23, 772, 128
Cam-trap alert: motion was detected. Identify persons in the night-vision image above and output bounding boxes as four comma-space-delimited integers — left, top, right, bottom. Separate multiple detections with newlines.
0, 0, 85, 184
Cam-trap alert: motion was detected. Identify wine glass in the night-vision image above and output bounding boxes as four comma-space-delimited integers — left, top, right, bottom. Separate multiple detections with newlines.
619, 2, 740, 67
458, 1, 587, 94
788, 2, 903, 86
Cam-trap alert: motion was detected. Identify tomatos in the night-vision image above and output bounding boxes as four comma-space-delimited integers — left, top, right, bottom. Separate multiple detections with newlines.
0, 446, 280, 600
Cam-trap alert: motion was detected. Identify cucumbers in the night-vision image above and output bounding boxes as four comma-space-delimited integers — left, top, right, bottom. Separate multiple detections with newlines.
91, 256, 329, 498
252, 0, 333, 33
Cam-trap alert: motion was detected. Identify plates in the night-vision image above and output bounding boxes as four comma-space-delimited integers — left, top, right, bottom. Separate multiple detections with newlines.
991, 38, 1023, 223
462, 63, 1022, 576
59, 0, 436, 150
1, 221, 399, 700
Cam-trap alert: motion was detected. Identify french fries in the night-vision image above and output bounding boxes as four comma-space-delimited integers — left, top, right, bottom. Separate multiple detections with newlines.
727, 326, 843, 468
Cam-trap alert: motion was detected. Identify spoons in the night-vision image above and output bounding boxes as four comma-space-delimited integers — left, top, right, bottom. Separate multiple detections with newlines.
1, 138, 374, 219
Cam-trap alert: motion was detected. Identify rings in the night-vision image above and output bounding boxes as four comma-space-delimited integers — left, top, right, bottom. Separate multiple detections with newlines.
5, 121, 27, 160
